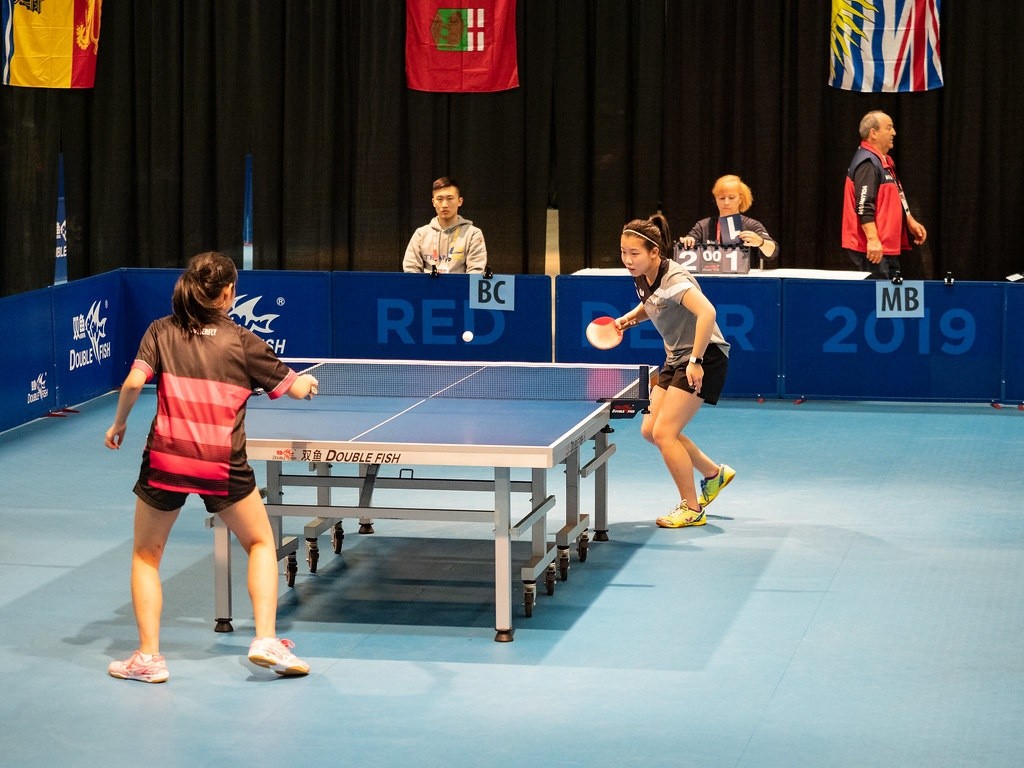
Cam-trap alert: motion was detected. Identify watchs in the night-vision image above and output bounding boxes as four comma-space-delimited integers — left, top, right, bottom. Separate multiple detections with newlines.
689, 355, 703, 364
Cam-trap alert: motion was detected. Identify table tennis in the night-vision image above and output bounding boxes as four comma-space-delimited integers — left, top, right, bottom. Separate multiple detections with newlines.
462, 331, 474, 342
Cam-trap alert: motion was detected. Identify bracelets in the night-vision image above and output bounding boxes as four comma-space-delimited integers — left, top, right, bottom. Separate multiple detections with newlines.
757, 238, 764, 248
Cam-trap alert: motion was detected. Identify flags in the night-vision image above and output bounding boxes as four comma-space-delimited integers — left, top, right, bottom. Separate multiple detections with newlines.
0, 0, 103, 89
404, 0, 520, 93
828, 0, 945, 93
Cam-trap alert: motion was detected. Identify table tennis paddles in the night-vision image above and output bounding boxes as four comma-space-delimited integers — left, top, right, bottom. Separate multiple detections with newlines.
586, 316, 638, 350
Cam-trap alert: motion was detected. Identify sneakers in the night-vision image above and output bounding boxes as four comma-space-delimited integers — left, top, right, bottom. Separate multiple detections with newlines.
248, 638, 310, 675
655, 499, 707, 528
698, 464, 736, 507
109, 651, 168, 683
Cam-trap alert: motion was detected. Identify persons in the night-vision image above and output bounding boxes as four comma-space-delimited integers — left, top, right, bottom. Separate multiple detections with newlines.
841, 111, 928, 281
102, 251, 318, 685
402, 176, 487, 274
613, 214, 735, 527
679, 174, 781, 269
